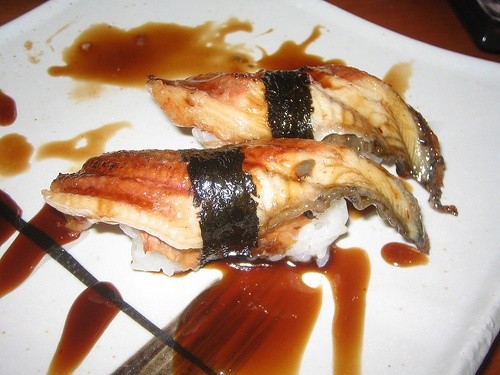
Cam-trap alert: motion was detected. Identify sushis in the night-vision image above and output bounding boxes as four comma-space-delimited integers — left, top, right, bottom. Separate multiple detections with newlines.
40, 61, 453, 277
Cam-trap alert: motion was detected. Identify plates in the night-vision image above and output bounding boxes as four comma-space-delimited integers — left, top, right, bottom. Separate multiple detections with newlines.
1, 0, 500, 375
